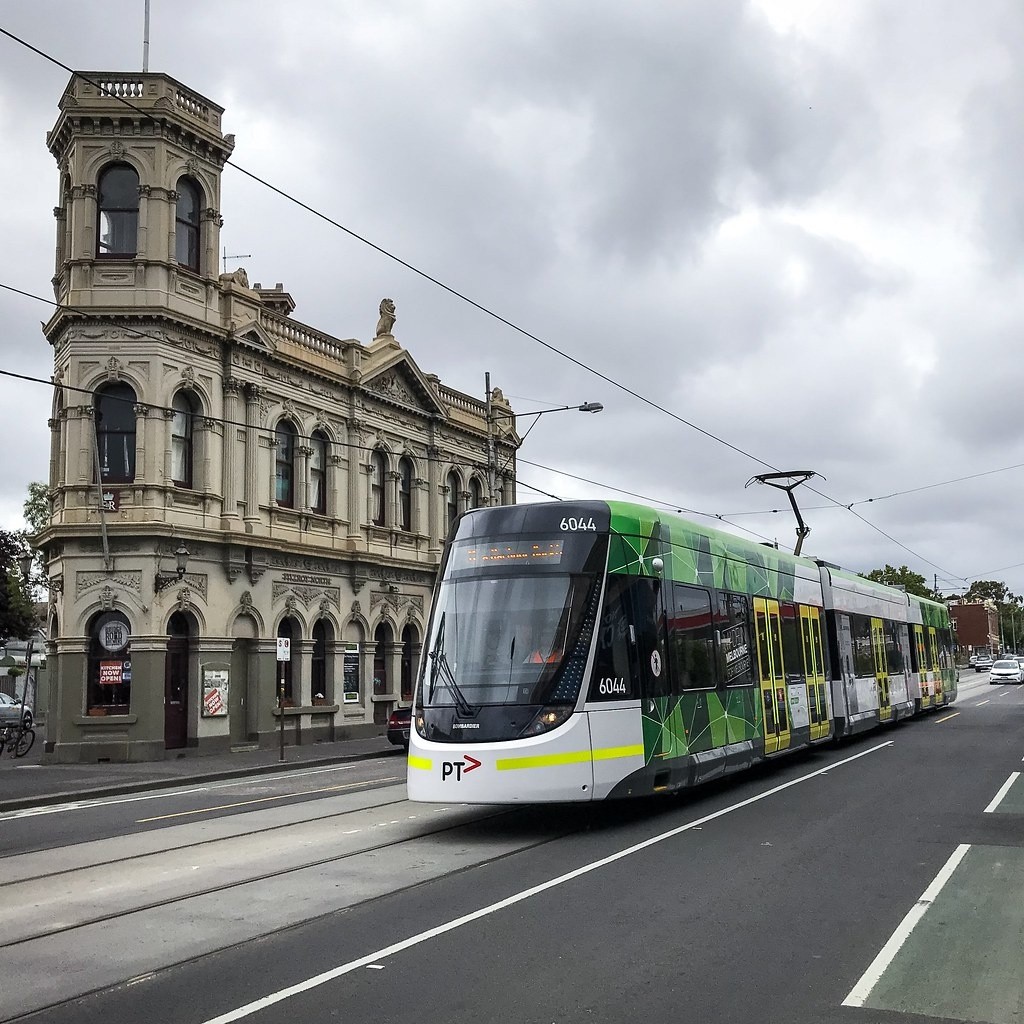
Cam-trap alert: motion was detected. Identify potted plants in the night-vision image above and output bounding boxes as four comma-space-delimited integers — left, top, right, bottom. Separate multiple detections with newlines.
8, 667, 25, 678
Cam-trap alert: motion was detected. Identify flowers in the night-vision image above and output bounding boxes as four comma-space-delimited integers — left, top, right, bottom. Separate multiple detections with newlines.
315, 693, 325, 699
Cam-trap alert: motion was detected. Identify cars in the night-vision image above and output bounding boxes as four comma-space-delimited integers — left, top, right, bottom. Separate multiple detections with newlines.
0, 641, 47, 667
0, 694, 35, 730
1013, 657, 1024, 671
1002, 654, 1017, 660
968, 656, 978, 667
955, 665, 959, 682
974, 654, 994, 672
989, 659, 1024, 685
386, 706, 412, 751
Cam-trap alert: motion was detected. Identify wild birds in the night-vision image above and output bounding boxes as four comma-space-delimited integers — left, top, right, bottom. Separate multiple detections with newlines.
314, 692, 324, 699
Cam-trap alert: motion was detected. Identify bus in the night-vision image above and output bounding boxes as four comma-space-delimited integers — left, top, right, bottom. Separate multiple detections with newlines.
406, 500, 957, 806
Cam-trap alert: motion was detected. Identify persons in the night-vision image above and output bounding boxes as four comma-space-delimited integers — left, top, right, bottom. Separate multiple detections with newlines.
523, 623, 564, 663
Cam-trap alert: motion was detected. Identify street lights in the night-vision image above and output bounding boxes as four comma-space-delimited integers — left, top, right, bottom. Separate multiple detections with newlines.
486, 403, 603, 506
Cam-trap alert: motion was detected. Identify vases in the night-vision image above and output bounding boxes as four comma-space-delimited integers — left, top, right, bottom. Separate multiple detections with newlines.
403, 695, 413, 701
89, 709, 109, 716
279, 701, 296, 707
312, 699, 329, 706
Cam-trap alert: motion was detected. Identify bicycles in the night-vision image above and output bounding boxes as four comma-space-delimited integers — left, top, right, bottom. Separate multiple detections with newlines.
0, 719, 35, 757
